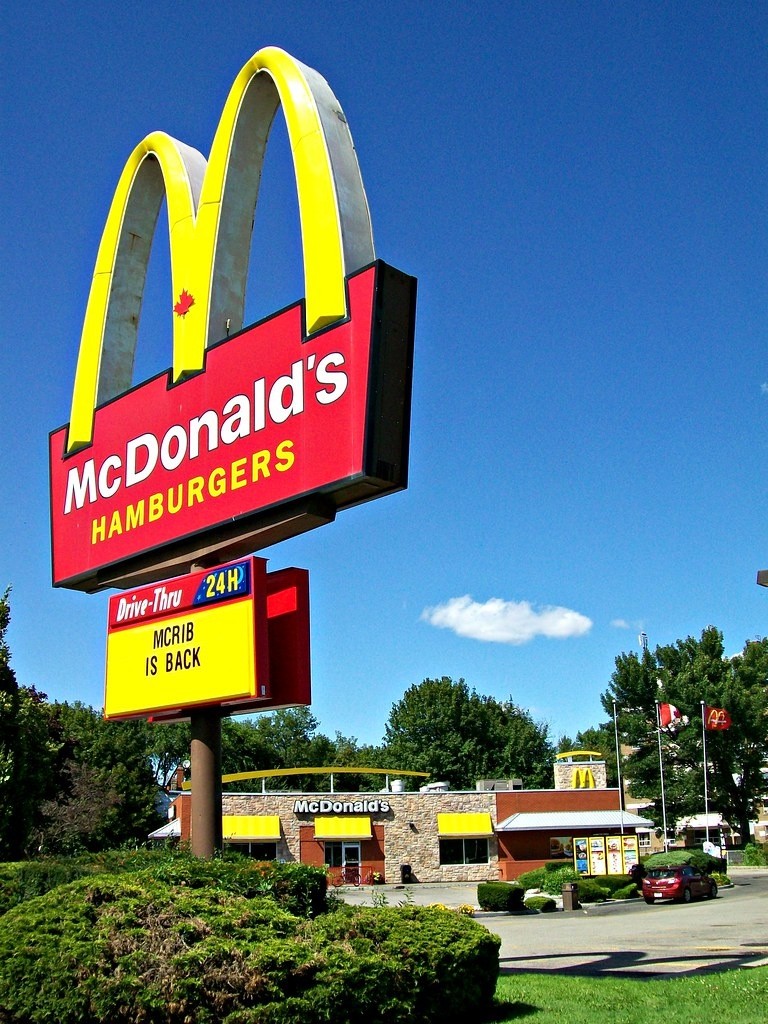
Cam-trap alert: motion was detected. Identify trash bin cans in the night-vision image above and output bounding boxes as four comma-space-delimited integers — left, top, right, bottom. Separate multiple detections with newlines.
561, 883, 579, 912
400, 864, 411, 884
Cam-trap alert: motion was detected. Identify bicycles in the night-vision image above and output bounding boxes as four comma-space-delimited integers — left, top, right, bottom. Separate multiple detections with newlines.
331, 867, 362, 888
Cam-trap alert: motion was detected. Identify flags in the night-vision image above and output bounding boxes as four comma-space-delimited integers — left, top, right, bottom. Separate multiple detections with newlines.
704, 706, 731, 730
658, 702, 682, 728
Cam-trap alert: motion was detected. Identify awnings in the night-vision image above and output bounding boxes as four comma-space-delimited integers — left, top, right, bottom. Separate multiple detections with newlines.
222, 815, 282, 843
312, 815, 373, 841
493, 809, 655, 832
147, 817, 182, 839
436, 812, 495, 839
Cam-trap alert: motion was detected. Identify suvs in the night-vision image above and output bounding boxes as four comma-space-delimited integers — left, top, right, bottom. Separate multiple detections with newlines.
641, 865, 718, 905
